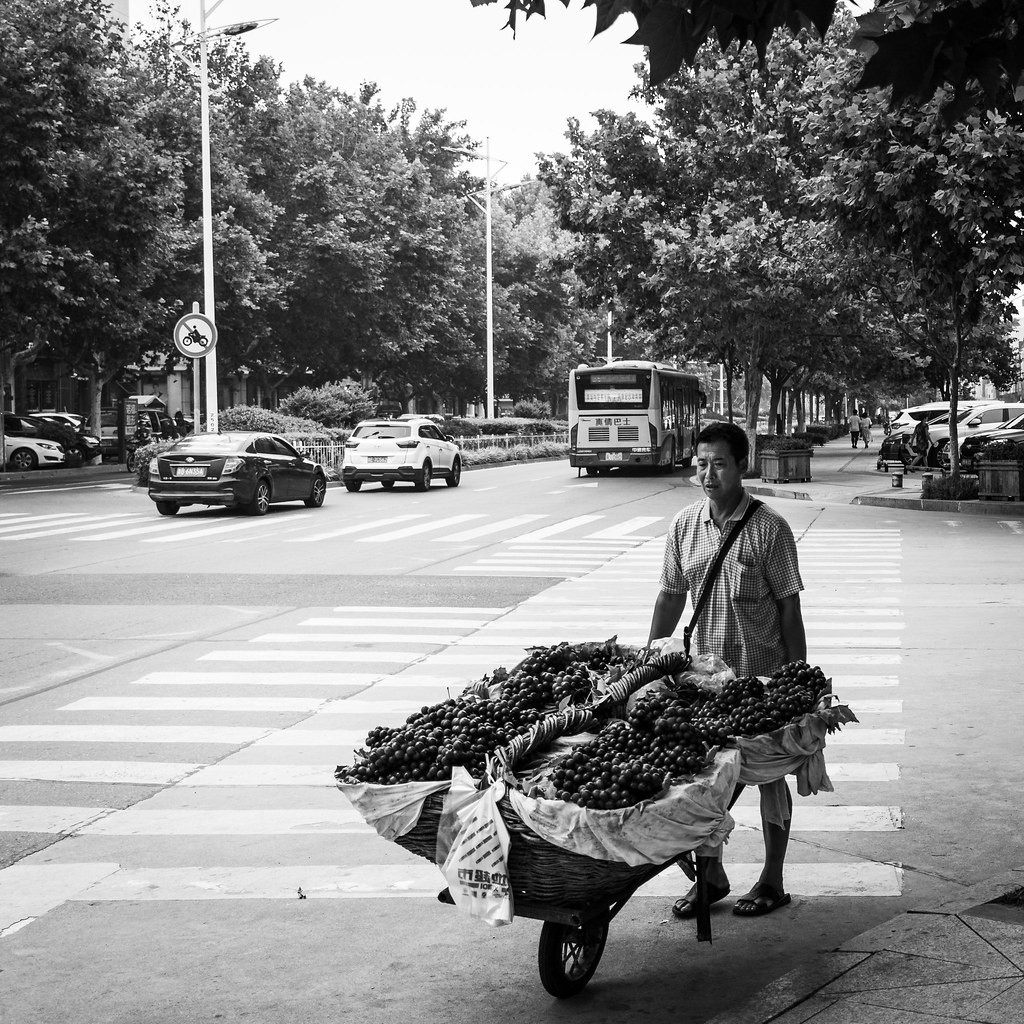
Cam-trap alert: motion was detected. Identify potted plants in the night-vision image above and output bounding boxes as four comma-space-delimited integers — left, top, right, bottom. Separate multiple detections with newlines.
757, 438, 815, 485
972, 439, 1024, 502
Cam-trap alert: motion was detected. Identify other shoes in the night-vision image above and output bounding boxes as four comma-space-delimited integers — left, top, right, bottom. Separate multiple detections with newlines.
908, 469, 916, 473
924, 468, 934, 472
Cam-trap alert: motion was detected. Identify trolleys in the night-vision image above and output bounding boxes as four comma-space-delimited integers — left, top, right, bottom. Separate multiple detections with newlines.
437, 780, 750, 1001
876, 431, 912, 476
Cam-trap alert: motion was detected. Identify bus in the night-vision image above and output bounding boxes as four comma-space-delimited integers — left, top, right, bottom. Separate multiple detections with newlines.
568, 360, 707, 477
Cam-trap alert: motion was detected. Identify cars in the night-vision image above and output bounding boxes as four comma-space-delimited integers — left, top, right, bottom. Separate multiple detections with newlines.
0, 409, 194, 472
397, 414, 445, 426
147, 430, 327, 514
887, 400, 1024, 477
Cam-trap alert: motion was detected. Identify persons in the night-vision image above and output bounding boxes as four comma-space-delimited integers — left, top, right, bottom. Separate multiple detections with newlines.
156, 418, 179, 443
149, 383, 163, 398
647, 422, 807, 919
174, 411, 194, 437
862, 412, 872, 449
876, 414, 882, 428
75, 417, 88, 433
849, 409, 862, 449
126, 422, 151, 468
909, 412, 934, 473
885, 414, 893, 428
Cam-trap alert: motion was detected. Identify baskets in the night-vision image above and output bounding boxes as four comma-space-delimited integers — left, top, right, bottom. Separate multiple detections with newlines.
336, 655, 690, 905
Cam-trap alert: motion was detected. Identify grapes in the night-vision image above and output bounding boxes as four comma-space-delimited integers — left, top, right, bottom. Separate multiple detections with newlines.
342, 643, 827, 806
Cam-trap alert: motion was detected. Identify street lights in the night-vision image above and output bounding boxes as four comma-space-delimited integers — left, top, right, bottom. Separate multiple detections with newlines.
440, 138, 542, 419
201, 16, 281, 434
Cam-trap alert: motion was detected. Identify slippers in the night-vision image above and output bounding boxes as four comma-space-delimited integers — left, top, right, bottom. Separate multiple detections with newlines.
672, 880, 731, 918
734, 881, 791, 916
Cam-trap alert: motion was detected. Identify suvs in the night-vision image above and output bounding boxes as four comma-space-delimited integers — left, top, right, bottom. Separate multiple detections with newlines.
340, 417, 463, 492
